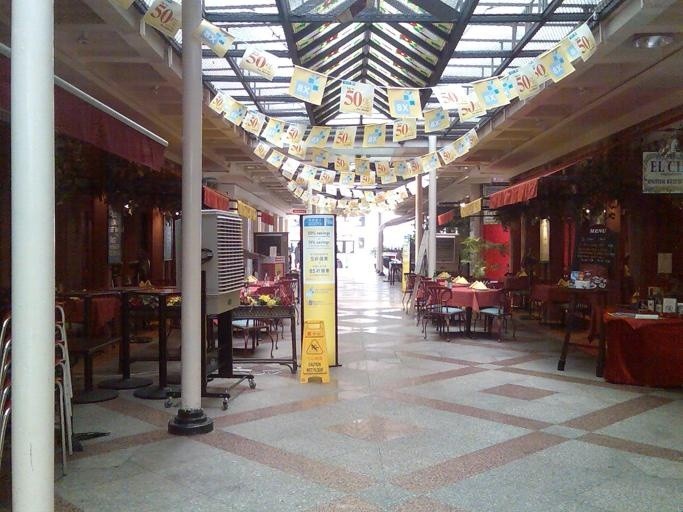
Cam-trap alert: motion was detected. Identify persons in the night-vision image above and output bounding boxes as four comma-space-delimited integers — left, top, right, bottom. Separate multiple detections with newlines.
292, 242, 300, 270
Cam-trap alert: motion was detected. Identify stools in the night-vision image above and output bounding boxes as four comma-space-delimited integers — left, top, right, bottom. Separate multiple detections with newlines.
0, 300, 74, 483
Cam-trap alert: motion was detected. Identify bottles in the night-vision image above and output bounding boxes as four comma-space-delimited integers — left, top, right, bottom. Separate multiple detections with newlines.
570, 270, 592, 289
259, 271, 279, 287
639, 288, 662, 313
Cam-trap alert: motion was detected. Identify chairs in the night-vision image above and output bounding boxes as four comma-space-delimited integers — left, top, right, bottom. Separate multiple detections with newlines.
553, 293, 608, 378
399, 270, 518, 342
214, 276, 301, 360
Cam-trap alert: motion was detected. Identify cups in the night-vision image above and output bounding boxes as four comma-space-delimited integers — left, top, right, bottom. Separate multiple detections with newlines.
269, 246, 277, 256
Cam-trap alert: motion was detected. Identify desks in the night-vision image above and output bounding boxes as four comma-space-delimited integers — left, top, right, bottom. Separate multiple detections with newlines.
95, 286, 154, 390
131, 289, 184, 398
63, 287, 120, 403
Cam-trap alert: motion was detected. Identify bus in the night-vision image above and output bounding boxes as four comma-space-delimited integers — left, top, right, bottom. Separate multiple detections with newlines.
288, 235, 357, 269
